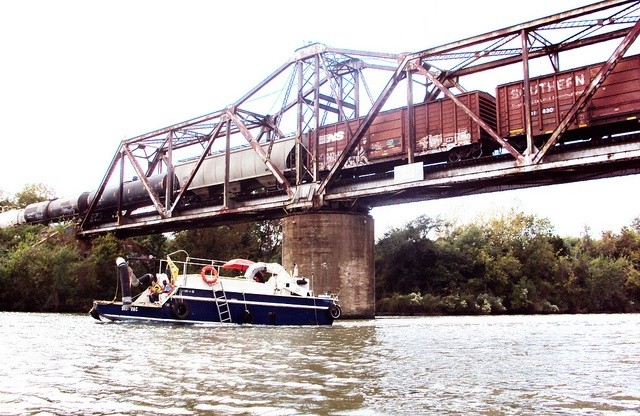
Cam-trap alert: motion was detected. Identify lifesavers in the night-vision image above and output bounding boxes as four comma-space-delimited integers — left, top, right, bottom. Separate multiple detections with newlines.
173, 302, 190, 319
328, 304, 342, 320
202, 265, 219, 284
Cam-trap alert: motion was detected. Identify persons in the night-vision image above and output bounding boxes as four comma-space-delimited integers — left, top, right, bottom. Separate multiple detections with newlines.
253, 270, 266, 283
147, 280, 162, 303
162, 280, 174, 295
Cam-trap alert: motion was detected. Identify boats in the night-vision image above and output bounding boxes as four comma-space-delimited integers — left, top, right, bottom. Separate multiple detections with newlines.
89, 249, 341, 328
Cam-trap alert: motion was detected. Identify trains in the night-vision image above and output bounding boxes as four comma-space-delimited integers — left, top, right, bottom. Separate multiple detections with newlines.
0, 52, 639, 229
